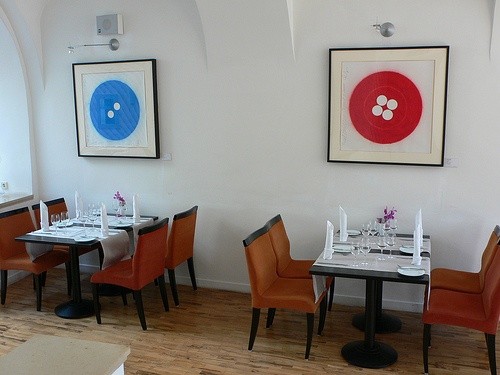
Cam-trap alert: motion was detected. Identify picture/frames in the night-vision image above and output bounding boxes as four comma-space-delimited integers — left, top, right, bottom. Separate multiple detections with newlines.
327, 46, 449, 167
72, 59, 160, 159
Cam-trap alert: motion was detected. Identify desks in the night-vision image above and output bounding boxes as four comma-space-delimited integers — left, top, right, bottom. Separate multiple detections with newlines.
308, 230, 431, 369
15, 214, 159, 319
0, 334, 130, 375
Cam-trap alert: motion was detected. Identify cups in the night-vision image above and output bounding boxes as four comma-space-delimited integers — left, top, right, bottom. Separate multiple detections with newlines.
420, 239, 424, 248
324, 248, 333, 259
40, 222, 49, 231
340, 233, 347, 242
101, 228, 109, 237
413, 257, 422, 265
133, 216, 141, 223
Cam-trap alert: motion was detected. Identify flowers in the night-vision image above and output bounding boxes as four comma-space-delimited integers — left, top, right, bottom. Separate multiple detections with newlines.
384, 206, 397, 222
113, 191, 126, 202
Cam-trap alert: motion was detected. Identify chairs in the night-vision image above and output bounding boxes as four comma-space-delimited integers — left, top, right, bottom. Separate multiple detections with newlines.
90, 217, 170, 330
132, 206, 199, 306
242, 214, 335, 359
423, 224, 500, 375
0, 197, 104, 311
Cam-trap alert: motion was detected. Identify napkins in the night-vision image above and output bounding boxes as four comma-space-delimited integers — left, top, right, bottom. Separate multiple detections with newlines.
40, 200, 49, 231
412, 209, 423, 265
339, 204, 348, 242
101, 202, 109, 236
75, 191, 81, 218
324, 220, 334, 260
133, 193, 140, 223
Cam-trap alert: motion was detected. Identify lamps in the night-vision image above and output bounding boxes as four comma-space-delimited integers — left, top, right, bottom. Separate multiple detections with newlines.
373, 23, 395, 37
68, 39, 119, 54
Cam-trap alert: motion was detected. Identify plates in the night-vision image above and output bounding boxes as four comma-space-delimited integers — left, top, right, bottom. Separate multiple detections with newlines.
399, 246, 423, 254
397, 266, 425, 277
333, 244, 354, 253
55, 222, 73, 227
74, 234, 97, 242
109, 220, 132, 227
346, 229, 361, 236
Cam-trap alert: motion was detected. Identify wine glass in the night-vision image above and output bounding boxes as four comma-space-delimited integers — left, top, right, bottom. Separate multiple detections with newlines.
351, 241, 361, 266
76, 200, 128, 224
51, 213, 60, 236
386, 232, 397, 260
377, 235, 386, 261
61, 211, 70, 235
88, 209, 97, 232
78, 211, 89, 237
360, 217, 398, 244
359, 237, 372, 265
